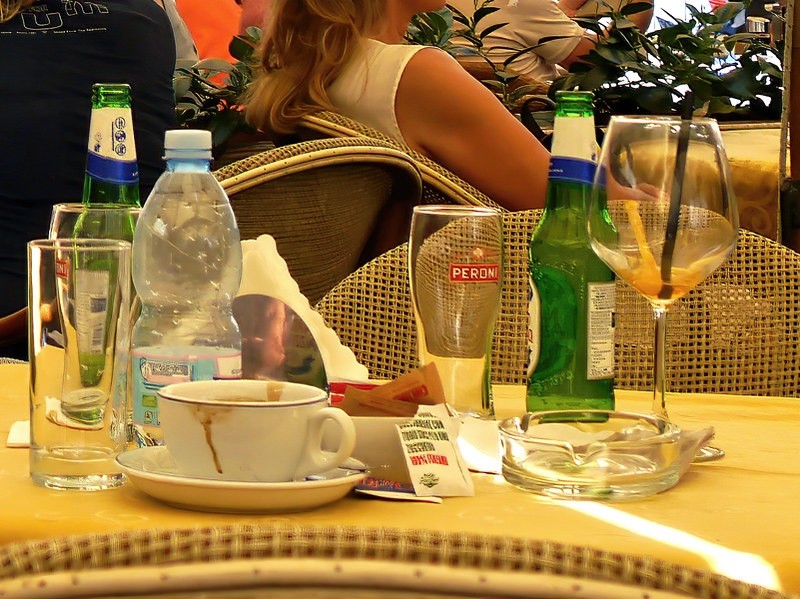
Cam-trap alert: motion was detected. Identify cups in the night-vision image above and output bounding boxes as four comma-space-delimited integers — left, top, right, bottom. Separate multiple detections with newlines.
156, 379, 358, 481
409, 205, 503, 420
49, 202, 145, 242
27, 239, 132, 490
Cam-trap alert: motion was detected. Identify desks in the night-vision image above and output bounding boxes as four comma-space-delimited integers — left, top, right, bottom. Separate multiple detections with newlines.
0, 359, 800, 599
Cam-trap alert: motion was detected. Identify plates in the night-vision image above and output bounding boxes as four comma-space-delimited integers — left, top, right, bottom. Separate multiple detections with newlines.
115, 447, 371, 514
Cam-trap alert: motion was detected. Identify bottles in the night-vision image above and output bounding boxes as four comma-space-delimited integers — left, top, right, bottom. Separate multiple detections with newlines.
83, 83, 142, 208
132, 129, 243, 447
524, 90, 620, 413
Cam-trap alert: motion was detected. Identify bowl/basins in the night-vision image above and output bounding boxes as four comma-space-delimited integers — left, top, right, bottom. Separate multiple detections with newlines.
322, 416, 459, 494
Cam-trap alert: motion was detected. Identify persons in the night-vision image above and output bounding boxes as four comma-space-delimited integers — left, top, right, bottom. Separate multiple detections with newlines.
243, 0, 670, 218
439, 0, 654, 101
2, 0, 201, 365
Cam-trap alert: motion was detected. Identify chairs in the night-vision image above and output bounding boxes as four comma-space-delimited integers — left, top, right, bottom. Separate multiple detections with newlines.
158, 107, 800, 399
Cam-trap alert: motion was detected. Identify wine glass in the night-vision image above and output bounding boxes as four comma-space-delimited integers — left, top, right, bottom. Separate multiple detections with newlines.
586, 114, 739, 463
